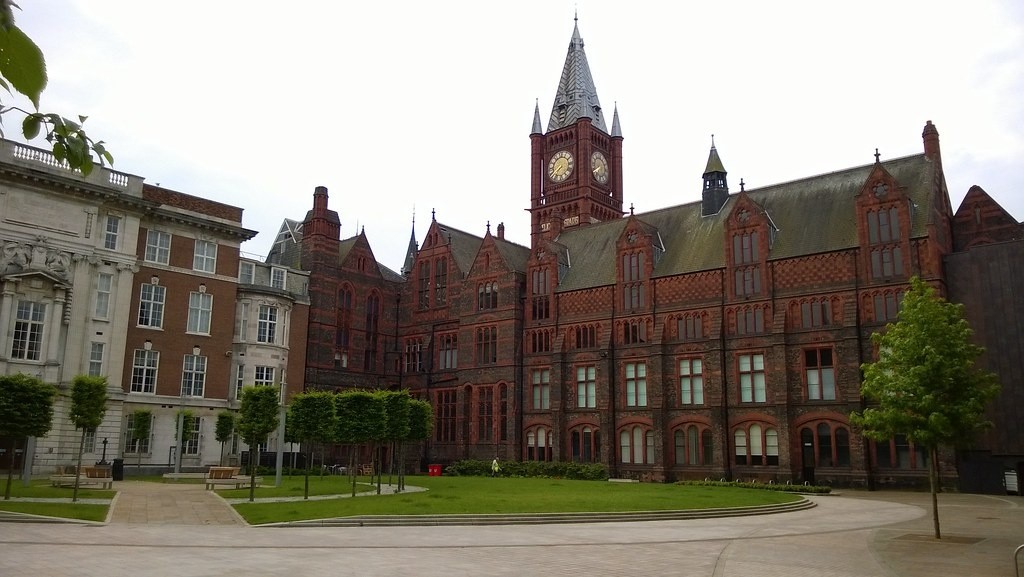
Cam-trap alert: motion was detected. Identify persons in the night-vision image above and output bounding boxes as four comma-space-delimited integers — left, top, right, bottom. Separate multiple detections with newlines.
491, 457, 502, 478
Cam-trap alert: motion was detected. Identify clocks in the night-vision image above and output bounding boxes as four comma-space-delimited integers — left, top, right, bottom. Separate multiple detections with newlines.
548, 151, 575, 183
591, 152, 610, 184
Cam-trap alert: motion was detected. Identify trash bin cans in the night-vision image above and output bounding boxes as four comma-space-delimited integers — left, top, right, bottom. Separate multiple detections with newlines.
427, 463, 442, 476
112, 458, 125, 481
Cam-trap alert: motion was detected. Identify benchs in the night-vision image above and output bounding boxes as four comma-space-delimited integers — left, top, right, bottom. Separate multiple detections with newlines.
206, 466, 263, 490
49, 465, 113, 488
